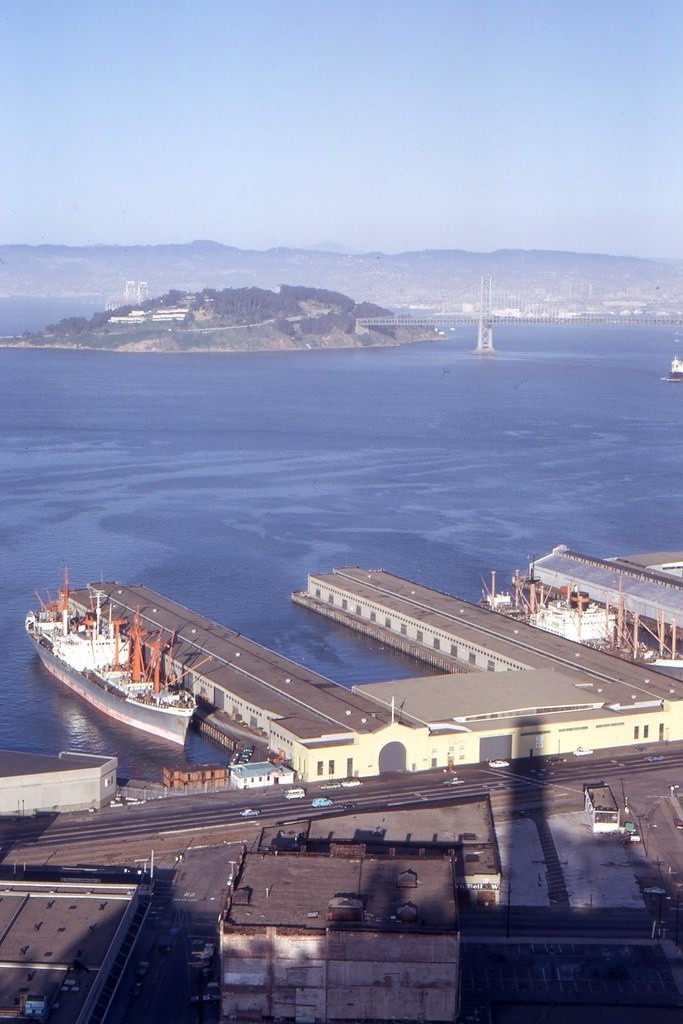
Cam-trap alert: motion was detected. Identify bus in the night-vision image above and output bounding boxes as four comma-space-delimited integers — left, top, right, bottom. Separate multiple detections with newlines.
284, 787, 305, 800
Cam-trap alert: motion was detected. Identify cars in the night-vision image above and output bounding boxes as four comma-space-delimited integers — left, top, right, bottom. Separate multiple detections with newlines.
443, 777, 465, 787
320, 781, 342, 790
339, 801, 357, 809
573, 747, 593, 757
674, 819, 683, 830
488, 760, 510, 768
312, 798, 334, 808
239, 808, 260, 817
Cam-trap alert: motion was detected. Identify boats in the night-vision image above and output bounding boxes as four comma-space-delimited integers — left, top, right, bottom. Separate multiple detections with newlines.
24, 566, 213, 746
478, 550, 683, 668
